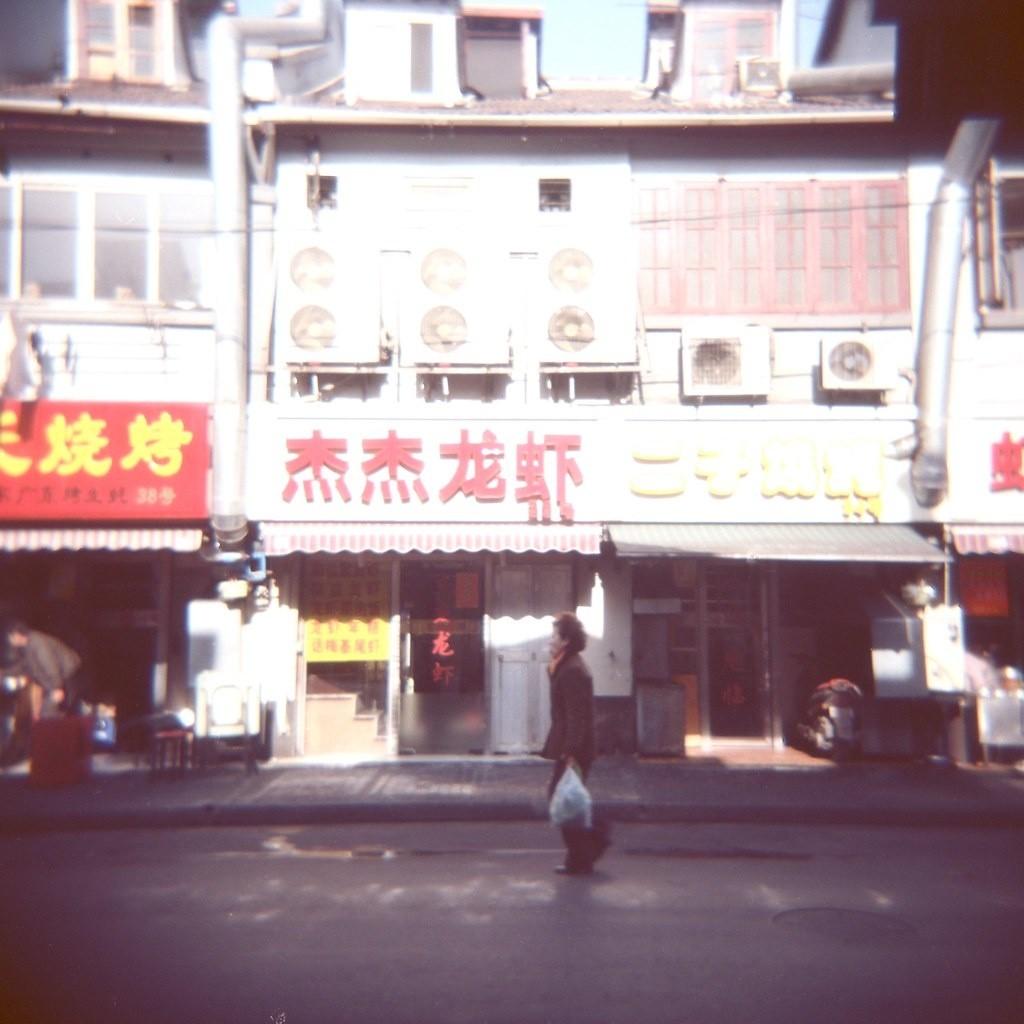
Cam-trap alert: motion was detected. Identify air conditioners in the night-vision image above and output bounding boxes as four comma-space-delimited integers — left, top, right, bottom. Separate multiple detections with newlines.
679, 324, 775, 397
272, 234, 636, 368
821, 334, 901, 389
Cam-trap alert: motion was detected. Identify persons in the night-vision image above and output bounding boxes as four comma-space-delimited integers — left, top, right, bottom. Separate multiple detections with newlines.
5, 623, 80, 726
541, 616, 609, 878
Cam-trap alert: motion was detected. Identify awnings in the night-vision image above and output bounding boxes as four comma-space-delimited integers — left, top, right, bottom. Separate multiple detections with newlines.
610, 523, 952, 563
943, 524, 1024, 554
2, 526, 203, 553
257, 523, 605, 556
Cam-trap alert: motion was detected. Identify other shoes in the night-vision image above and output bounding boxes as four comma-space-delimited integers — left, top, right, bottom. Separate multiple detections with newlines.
555, 865, 592, 875
587, 836, 612, 868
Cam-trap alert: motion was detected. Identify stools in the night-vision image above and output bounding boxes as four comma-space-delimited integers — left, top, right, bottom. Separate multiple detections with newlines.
155, 730, 190, 773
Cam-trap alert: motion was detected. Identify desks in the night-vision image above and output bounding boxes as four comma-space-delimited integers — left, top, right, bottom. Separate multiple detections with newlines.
191, 668, 272, 779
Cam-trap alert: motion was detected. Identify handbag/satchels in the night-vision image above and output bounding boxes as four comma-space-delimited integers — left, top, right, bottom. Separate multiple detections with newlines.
548, 766, 594, 831
